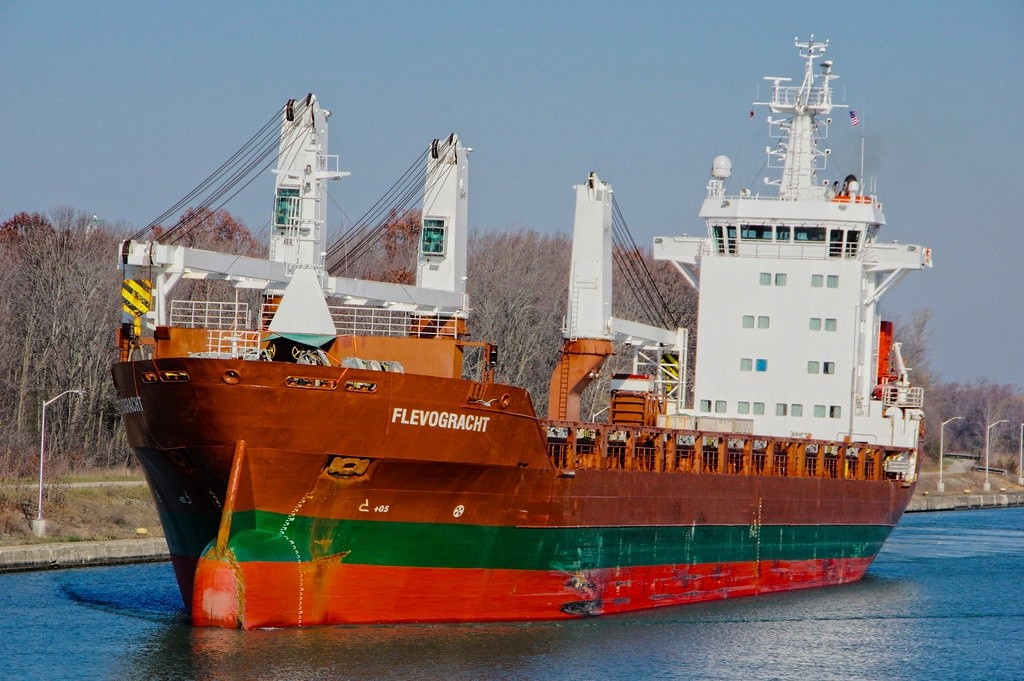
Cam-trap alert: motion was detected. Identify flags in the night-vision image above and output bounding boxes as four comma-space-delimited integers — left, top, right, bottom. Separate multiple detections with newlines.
850, 111, 860, 125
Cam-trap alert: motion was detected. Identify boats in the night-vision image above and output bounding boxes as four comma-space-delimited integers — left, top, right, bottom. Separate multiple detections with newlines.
110, 34, 931, 630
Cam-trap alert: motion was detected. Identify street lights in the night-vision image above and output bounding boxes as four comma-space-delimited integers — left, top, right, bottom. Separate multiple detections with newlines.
1017, 422, 1023, 485
32, 390, 86, 537
937, 417, 965, 492
984, 420, 1010, 490
